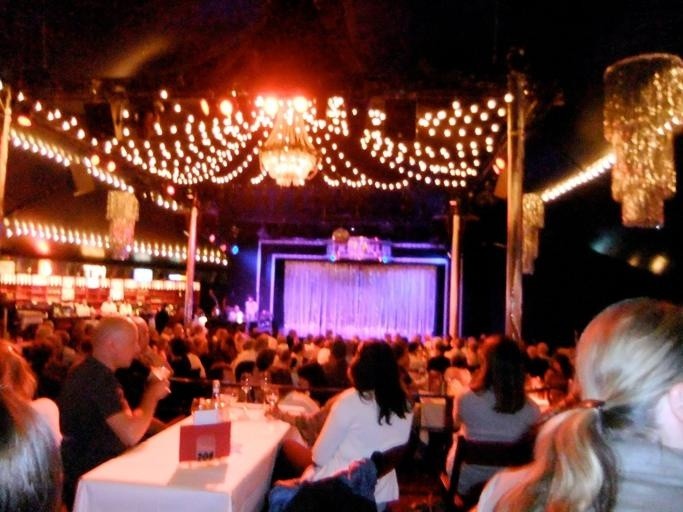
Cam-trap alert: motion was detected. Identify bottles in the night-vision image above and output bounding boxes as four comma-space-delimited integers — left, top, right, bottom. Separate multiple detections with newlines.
210, 378, 221, 410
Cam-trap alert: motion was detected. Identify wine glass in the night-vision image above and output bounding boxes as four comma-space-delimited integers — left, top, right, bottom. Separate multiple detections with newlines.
237, 370, 281, 411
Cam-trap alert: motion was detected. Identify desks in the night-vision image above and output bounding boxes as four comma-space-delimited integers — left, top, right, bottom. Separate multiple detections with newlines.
71, 401, 304, 512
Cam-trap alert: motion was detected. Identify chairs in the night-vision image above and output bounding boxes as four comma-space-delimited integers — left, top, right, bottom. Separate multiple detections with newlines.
438, 433, 533, 512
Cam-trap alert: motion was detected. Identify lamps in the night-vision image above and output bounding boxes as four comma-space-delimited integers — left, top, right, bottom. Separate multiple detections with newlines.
519, 192, 546, 275
258, 109, 318, 189
105, 190, 139, 262
599, 53, 683, 231
